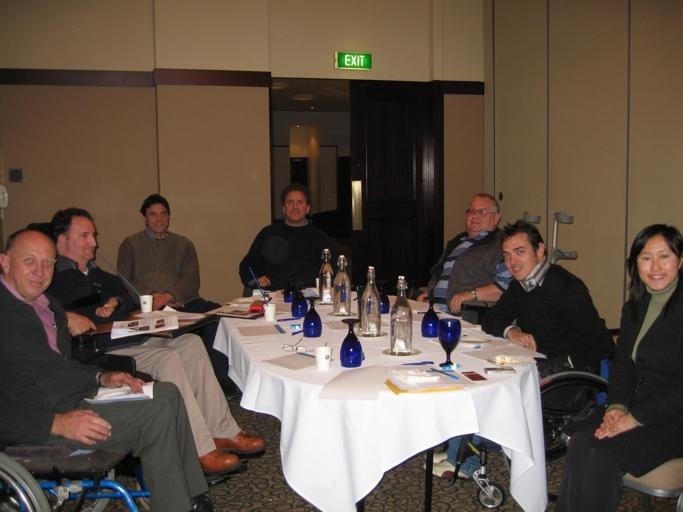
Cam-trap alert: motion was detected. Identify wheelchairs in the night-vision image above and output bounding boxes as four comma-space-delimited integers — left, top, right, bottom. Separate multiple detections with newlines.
0, 434, 151, 512
452, 317, 621, 510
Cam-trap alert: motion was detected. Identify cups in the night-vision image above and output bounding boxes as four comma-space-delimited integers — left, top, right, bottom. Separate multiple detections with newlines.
263, 305, 277, 322
139, 294, 154, 313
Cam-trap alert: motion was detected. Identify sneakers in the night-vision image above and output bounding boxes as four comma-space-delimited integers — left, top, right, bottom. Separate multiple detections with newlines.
421, 459, 471, 482
214, 432, 267, 453
199, 451, 242, 476
422, 453, 448, 463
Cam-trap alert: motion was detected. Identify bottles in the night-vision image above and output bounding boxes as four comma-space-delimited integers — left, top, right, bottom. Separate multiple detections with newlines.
318, 248, 413, 356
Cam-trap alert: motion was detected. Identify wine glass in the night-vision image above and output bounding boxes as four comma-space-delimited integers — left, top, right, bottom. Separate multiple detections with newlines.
279, 272, 461, 374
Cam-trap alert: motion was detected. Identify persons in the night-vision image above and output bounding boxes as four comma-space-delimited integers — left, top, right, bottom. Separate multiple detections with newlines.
553, 223, 683, 512
117, 193, 242, 399
238, 183, 339, 297
45, 208, 265, 475
0, 227, 217, 512
416, 194, 514, 328
422, 218, 601, 480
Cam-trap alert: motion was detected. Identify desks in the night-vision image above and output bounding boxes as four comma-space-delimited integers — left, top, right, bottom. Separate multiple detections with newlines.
212, 281, 549, 512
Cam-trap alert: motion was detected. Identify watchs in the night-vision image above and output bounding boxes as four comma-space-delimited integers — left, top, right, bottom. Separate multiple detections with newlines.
469, 289, 477, 300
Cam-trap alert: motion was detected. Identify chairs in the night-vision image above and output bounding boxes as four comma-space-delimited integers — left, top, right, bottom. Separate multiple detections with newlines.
621, 456, 683, 512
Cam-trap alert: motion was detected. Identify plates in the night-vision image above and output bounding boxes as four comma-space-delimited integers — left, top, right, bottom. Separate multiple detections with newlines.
485, 355, 532, 368
